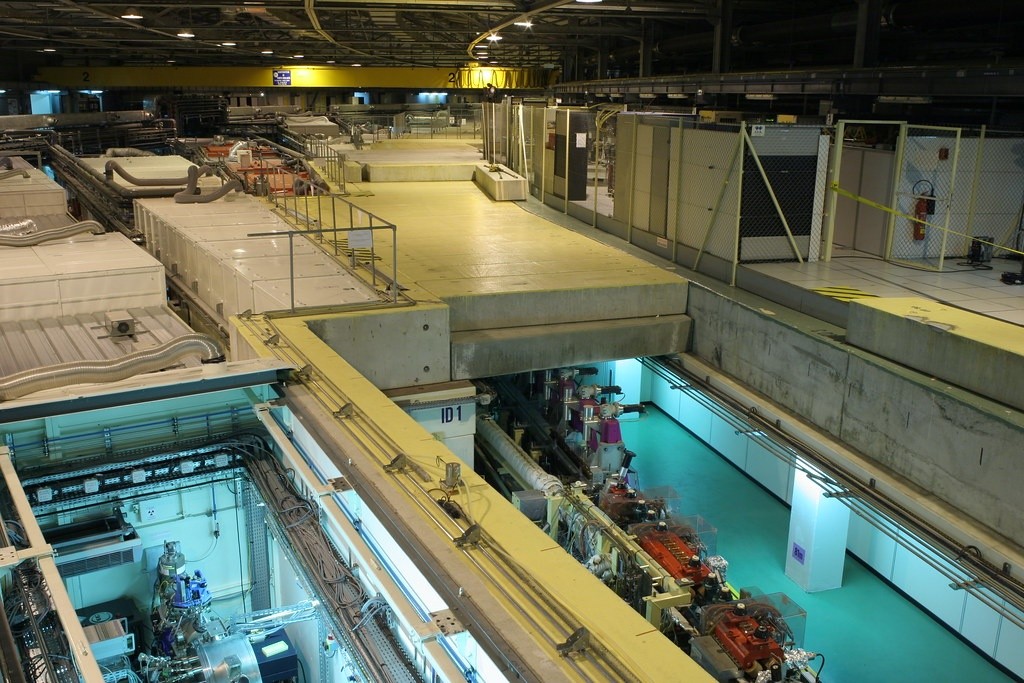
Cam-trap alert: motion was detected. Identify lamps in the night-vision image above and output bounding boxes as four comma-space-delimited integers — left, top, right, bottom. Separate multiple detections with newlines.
121, 6, 143, 19
177, 21, 195, 37
475, 21, 533, 64
222, 42, 362, 67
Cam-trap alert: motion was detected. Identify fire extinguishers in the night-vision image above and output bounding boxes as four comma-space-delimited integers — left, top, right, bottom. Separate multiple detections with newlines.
912, 180, 937, 240
